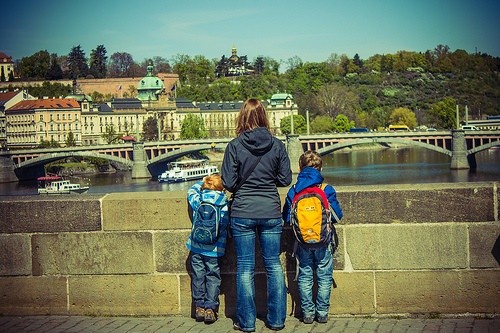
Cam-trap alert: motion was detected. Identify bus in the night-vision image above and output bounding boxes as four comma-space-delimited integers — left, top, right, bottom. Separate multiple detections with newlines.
350, 128, 369, 132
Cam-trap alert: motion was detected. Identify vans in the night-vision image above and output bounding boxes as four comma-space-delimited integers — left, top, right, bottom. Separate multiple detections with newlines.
462, 125, 480, 131
388, 124, 411, 132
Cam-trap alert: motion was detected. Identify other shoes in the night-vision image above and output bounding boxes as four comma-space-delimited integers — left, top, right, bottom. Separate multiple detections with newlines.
267, 313, 283, 329
204, 309, 217, 321
195, 307, 205, 320
318, 314, 328, 322
234, 320, 254, 331
303, 317, 315, 323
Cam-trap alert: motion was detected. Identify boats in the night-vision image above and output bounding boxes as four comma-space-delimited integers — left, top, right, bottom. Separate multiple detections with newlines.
37, 176, 90, 196
157, 158, 220, 184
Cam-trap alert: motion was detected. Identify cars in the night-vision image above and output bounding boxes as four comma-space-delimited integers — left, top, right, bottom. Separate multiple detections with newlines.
426, 127, 437, 132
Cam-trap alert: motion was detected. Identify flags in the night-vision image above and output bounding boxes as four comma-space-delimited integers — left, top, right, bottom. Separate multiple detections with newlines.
117, 85, 121, 90
171, 85, 175, 91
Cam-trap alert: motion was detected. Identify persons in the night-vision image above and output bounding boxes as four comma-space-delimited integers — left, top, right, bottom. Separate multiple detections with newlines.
221, 98, 293, 332
186, 173, 234, 323
282, 150, 342, 324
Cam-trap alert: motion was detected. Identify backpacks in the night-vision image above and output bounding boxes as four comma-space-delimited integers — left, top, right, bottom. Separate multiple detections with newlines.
191, 204, 225, 244
290, 184, 331, 248
